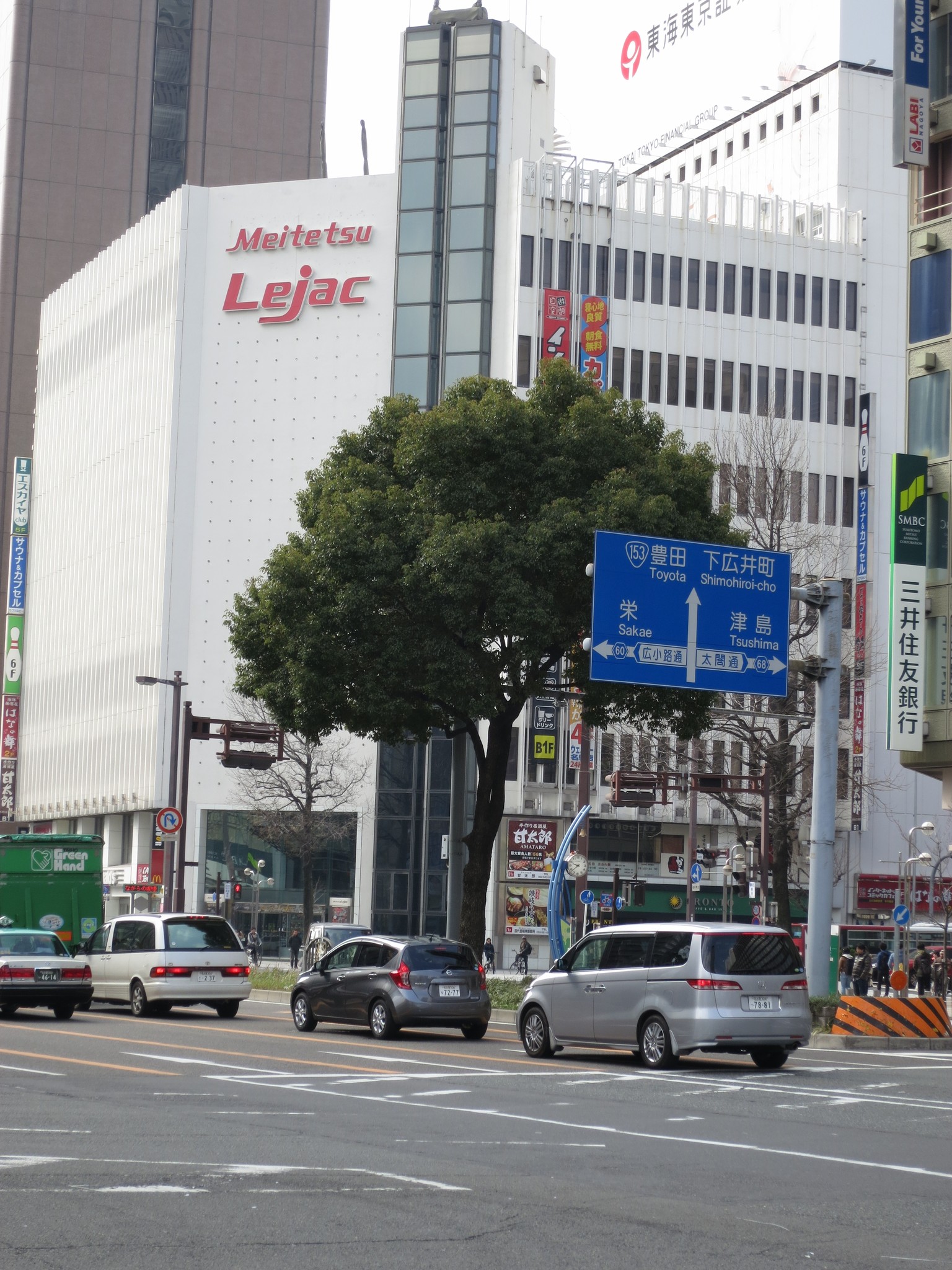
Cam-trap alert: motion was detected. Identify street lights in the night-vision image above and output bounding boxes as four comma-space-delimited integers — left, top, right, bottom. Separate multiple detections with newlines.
899, 852, 932, 998
722, 840, 754, 923
135, 670, 189, 913
906, 821, 952, 1012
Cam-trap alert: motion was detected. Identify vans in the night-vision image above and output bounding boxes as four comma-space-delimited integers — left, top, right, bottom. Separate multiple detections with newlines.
304, 920, 373, 959
515, 921, 814, 1071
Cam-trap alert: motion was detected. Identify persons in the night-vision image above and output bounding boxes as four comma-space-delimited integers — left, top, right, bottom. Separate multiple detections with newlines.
840, 942, 950, 1000
519, 936, 531, 976
237, 926, 302, 969
483, 938, 496, 976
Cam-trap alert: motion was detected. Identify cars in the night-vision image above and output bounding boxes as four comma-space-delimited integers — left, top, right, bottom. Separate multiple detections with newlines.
70, 911, 252, 1018
888, 944, 951, 988
0, 928, 95, 1020
290, 933, 492, 1040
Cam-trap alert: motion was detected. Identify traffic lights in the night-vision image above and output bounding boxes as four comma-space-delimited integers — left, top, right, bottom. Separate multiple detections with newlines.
696, 848, 721, 858
732, 870, 746, 897
233, 884, 244, 903
696, 857, 717, 868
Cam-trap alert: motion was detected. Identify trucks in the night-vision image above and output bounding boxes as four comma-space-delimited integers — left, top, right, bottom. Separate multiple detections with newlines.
0, 833, 106, 955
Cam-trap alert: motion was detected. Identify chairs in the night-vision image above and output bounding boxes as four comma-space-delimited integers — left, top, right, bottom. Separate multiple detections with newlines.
15, 938, 32, 952
615, 945, 645, 966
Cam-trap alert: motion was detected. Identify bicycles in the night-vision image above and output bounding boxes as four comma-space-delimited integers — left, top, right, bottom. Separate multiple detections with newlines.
246, 942, 262, 968
509, 949, 526, 975
484, 951, 499, 974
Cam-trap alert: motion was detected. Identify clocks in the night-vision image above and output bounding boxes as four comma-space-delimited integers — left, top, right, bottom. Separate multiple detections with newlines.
566, 851, 589, 877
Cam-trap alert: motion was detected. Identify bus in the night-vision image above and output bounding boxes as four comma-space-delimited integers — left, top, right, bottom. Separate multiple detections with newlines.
792, 922, 952, 991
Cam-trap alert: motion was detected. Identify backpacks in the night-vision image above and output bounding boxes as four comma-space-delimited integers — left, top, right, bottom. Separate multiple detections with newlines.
920, 957, 931, 975
842, 956, 854, 976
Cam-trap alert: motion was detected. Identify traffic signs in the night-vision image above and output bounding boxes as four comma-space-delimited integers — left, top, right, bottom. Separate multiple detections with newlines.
587, 529, 792, 699
